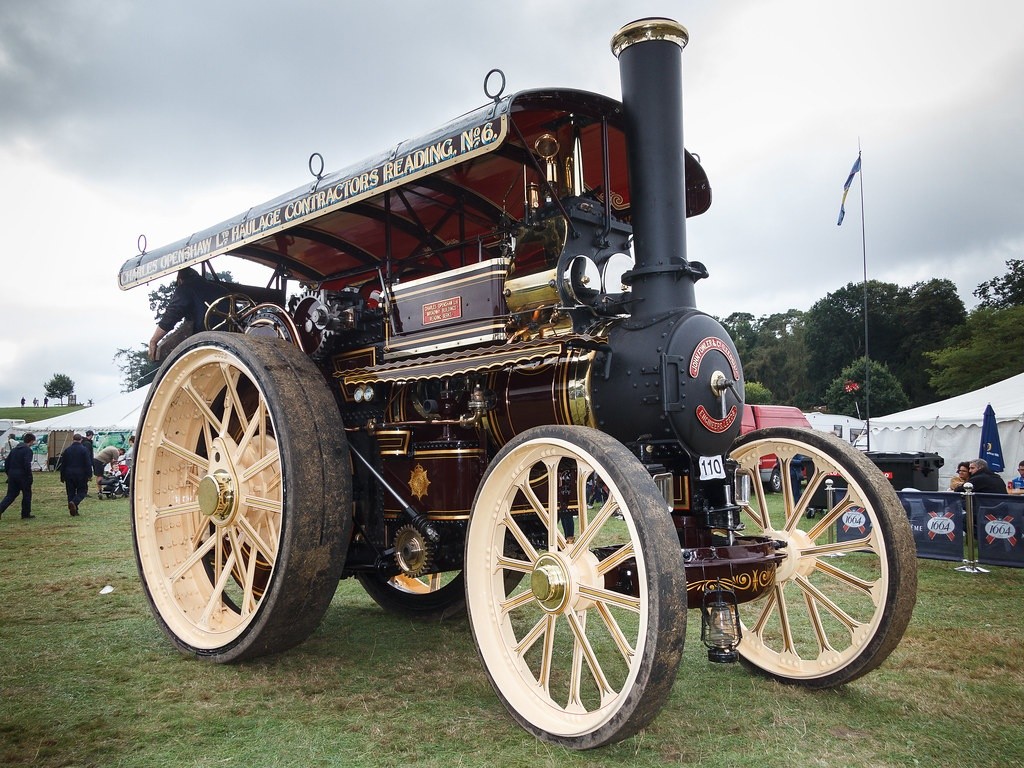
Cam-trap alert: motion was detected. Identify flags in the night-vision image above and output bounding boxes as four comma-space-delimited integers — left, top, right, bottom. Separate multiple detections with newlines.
838, 157, 861, 226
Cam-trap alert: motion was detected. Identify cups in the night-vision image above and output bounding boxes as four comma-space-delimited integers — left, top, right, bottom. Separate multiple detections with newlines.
1015, 482, 1021, 493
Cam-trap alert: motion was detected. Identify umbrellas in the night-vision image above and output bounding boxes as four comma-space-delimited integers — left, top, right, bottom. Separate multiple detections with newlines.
979, 401, 1005, 473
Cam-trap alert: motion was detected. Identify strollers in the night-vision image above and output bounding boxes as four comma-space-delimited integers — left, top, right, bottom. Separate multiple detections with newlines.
97, 457, 132, 500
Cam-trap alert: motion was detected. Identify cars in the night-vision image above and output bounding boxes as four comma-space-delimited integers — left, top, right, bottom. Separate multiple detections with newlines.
113, 15, 919, 755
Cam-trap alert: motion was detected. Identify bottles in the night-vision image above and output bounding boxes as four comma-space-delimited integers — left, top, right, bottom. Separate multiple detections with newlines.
1008, 480, 1013, 494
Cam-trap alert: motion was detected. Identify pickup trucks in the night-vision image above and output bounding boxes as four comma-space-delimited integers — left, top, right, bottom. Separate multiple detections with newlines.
729, 403, 818, 493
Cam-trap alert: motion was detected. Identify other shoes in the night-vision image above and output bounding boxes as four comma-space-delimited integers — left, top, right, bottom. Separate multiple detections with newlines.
98, 493, 102, 499
588, 505, 594, 509
68, 501, 79, 516
22, 515, 35, 519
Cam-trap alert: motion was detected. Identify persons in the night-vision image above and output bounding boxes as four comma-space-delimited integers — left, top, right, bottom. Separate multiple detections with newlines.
33, 398, 39, 407
118, 436, 136, 498
81, 430, 94, 481
43, 397, 49, 407
148, 266, 234, 361
60, 433, 93, 516
949, 459, 1009, 540
1007, 460, 1024, 495
93, 446, 126, 497
111, 465, 122, 486
21, 397, 26, 407
0, 433, 37, 520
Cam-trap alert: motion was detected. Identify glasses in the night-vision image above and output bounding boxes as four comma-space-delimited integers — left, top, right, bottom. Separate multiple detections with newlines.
1017, 469, 1024, 471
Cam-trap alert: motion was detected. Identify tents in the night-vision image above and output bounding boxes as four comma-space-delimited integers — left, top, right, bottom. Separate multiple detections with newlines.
868, 372, 1024, 492
10, 381, 153, 433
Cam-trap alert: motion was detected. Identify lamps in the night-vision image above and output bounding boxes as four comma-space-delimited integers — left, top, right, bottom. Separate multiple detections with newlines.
700, 557, 743, 663
